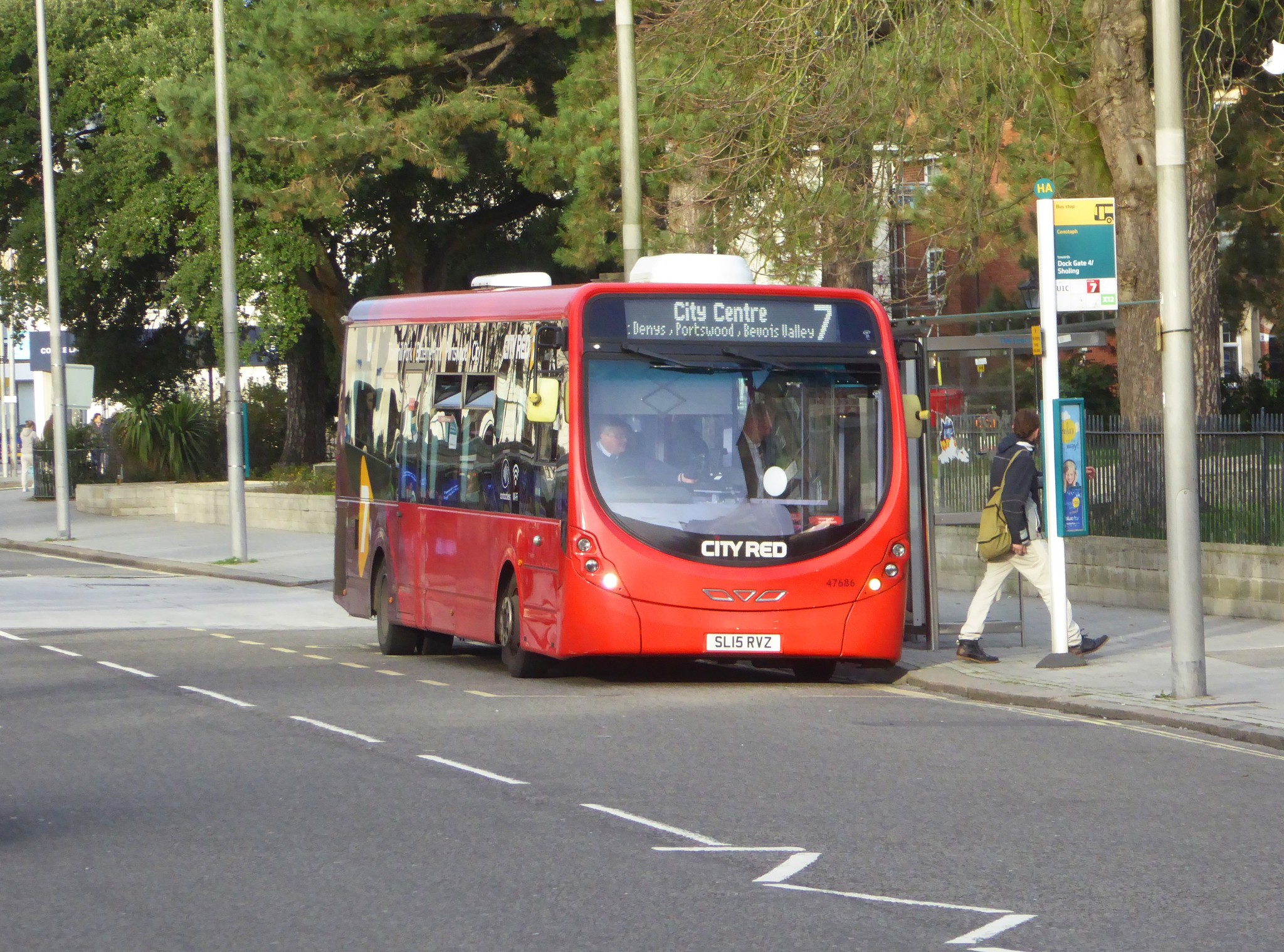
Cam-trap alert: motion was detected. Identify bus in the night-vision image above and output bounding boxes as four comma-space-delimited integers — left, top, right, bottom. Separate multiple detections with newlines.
331, 251, 913, 678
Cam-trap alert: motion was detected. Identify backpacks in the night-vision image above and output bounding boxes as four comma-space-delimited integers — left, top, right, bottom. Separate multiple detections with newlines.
977, 449, 1029, 562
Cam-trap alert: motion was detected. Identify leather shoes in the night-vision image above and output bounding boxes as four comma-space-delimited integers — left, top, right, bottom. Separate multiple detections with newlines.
957, 637, 999, 664
1068, 628, 1109, 657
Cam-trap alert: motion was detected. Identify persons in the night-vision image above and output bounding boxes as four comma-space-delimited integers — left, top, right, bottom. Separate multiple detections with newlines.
956, 409, 1109, 664
19, 413, 116, 491
1063, 459, 1079, 493
730, 404, 774, 499
592, 422, 698, 486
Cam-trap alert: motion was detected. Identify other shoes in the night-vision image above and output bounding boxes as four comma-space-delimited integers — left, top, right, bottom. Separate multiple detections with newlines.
23, 488, 30, 492
31, 485, 34, 489
